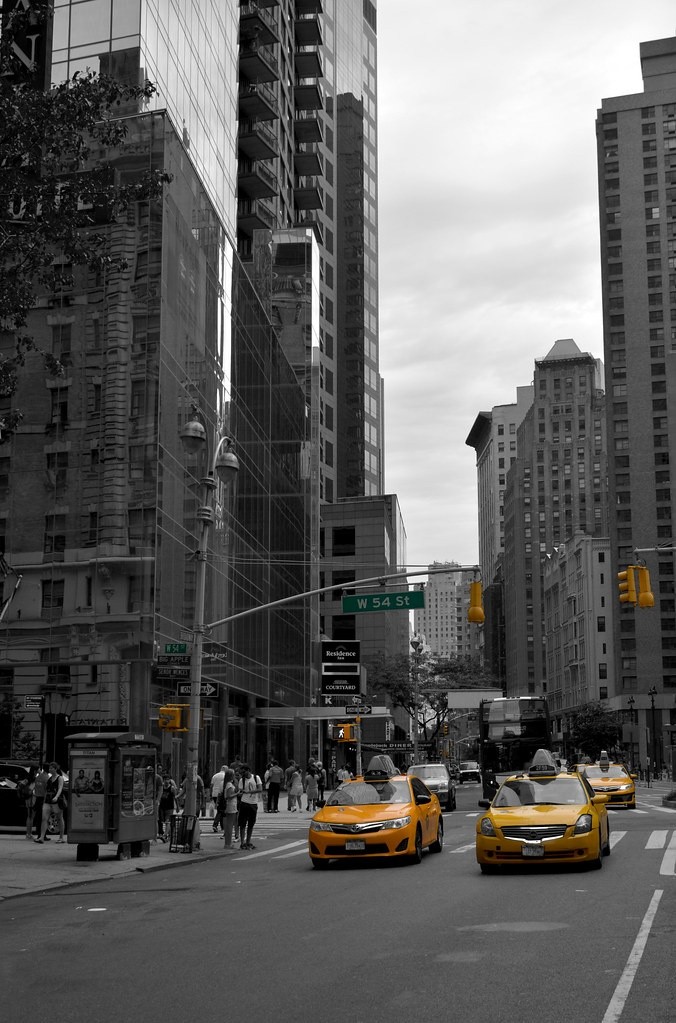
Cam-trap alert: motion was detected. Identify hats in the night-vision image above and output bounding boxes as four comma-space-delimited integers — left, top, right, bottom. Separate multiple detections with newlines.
309, 758, 315, 763
317, 761, 322, 766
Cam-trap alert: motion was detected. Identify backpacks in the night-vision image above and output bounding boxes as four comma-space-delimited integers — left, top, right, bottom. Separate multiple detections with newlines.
317, 768, 325, 784
218, 793, 226, 811
19, 778, 36, 802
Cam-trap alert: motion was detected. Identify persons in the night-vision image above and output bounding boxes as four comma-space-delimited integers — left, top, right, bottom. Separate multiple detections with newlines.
89, 770, 104, 794
156, 764, 205, 843
74, 770, 89, 798
209, 755, 262, 850
25, 762, 65, 843
336, 764, 354, 785
264, 757, 327, 813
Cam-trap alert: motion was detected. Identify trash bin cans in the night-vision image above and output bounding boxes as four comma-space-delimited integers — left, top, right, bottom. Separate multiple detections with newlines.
169, 815, 197, 852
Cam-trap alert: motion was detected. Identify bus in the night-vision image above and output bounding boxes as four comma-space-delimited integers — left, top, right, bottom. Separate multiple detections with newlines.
479, 697, 551, 802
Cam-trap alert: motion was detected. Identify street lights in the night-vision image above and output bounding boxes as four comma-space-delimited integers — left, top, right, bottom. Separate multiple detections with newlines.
647, 684, 658, 781
628, 694, 636, 786
181, 407, 242, 852
409, 632, 424, 763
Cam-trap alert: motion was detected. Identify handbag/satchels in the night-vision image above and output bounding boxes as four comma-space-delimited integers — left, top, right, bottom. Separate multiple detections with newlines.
209, 800, 214, 817
57, 793, 68, 810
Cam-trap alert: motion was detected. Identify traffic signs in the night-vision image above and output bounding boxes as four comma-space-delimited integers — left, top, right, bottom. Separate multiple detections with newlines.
177, 681, 220, 697
346, 705, 373, 717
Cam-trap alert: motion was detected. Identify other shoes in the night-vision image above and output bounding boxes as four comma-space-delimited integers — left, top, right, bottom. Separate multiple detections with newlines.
213, 826, 218, 833
220, 835, 224, 839
265, 809, 279, 813
291, 807, 296, 812
300, 809, 302, 812
34, 839, 44, 844
38, 835, 51, 841
288, 808, 291, 811
26, 834, 37, 840
56, 838, 65, 844
240, 844, 248, 850
234, 837, 240, 842
160, 835, 168, 843
306, 808, 309, 811
249, 843, 255, 849
313, 808, 316, 810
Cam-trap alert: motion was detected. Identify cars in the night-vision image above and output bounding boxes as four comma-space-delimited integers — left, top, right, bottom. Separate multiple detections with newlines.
0, 764, 69, 835
308, 755, 443, 868
403, 760, 481, 811
476, 749, 610, 875
568, 750, 637, 809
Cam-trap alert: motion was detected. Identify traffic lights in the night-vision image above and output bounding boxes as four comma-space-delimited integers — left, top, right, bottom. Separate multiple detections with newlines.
329, 723, 360, 743
442, 722, 449, 737
616, 565, 637, 611
